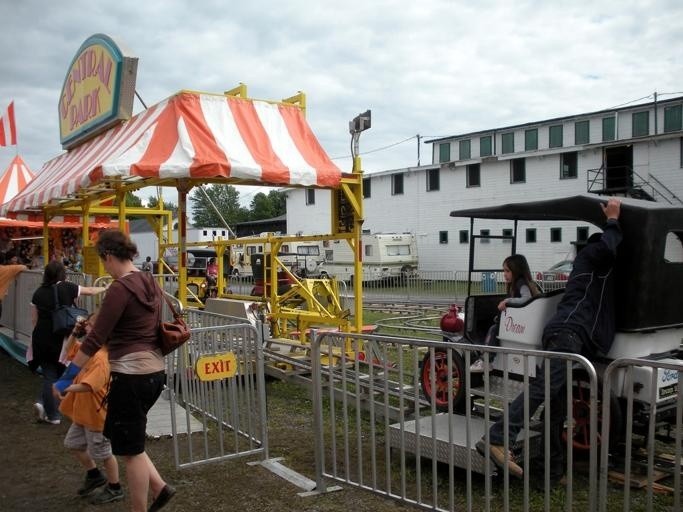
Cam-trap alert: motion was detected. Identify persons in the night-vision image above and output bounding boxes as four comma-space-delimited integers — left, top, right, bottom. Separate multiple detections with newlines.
470, 254, 546, 374
291, 265, 303, 279
206, 257, 218, 285
474, 196, 624, 486
189, 259, 204, 276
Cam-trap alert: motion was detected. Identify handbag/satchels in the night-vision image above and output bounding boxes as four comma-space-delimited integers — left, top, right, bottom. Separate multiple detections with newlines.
52, 304, 88, 336
159, 315, 191, 356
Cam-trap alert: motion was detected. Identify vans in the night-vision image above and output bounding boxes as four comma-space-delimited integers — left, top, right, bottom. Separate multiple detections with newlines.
317, 231, 417, 284
227, 233, 324, 281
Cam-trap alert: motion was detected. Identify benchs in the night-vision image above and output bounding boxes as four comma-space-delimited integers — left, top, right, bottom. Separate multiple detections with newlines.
492, 285, 566, 379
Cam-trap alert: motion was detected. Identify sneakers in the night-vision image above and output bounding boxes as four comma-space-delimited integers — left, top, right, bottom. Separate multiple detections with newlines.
34, 402, 60, 424
539, 471, 575, 491
475, 437, 523, 478
147, 484, 176, 512
77, 472, 106, 495
96, 485, 124, 504
471, 359, 493, 373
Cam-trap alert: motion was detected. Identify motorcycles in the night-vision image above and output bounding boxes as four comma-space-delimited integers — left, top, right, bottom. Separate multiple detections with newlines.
419, 188, 683, 455
249, 249, 332, 313
158, 245, 233, 302
